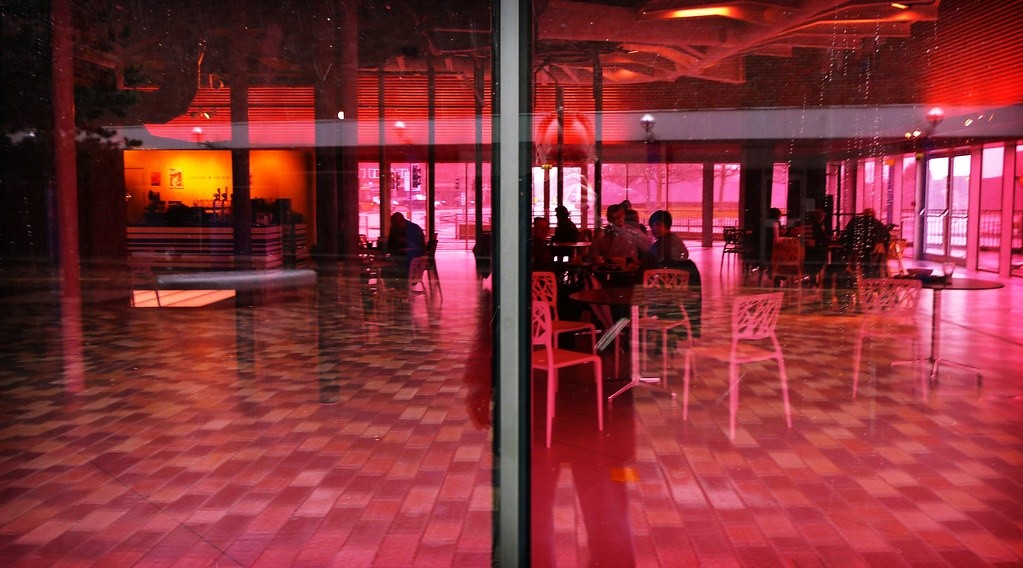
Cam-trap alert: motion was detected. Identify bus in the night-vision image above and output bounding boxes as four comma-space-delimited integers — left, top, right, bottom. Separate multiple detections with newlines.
359, 186, 375, 211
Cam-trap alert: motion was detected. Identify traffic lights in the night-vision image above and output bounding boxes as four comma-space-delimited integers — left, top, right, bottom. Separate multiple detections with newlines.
455, 175, 460, 190
410, 163, 421, 191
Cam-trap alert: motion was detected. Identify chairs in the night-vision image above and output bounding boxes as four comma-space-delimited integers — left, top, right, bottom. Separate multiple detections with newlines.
848, 277, 931, 401
628, 269, 699, 388
679, 292, 792, 441
530, 270, 624, 448
385, 233, 442, 337
719, 226, 752, 279
771, 238, 824, 313
126, 253, 161, 311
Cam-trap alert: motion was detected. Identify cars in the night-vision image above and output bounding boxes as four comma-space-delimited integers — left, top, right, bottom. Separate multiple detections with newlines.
402, 193, 427, 209
372, 196, 400, 209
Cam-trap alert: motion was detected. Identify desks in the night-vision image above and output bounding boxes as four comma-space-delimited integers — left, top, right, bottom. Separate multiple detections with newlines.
567, 289, 701, 401
545, 242, 593, 264
359, 246, 399, 327
574, 263, 641, 281
893, 275, 1005, 382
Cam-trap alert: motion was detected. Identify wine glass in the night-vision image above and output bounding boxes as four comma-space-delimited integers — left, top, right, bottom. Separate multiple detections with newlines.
943, 257, 956, 285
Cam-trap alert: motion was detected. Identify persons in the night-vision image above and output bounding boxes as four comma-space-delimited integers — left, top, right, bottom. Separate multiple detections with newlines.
388, 212, 425, 271
757, 208, 891, 316
594, 200, 702, 338
530, 206, 580, 269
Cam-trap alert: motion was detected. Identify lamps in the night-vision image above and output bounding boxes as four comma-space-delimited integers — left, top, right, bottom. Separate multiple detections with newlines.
924, 108, 945, 141
189, 107, 203, 117
193, 127, 212, 149
204, 107, 216, 120
394, 120, 406, 137
639, 112, 655, 145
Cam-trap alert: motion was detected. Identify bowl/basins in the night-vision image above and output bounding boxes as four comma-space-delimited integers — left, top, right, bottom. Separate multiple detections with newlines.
906, 268, 934, 276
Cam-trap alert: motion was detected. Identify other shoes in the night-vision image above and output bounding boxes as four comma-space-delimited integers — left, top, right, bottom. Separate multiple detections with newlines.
839, 301, 849, 315
823, 300, 833, 312
